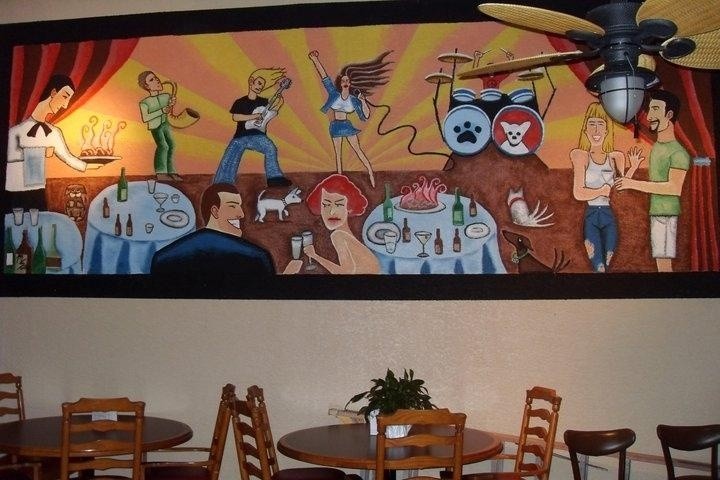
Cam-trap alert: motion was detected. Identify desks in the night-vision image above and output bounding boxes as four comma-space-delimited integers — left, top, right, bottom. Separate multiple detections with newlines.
0, 414, 193, 479
277, 423, 504, 480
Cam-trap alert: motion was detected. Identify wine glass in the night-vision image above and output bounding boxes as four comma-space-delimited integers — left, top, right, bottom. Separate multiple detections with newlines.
601, 170, 614, 199
303, 231, 316, 271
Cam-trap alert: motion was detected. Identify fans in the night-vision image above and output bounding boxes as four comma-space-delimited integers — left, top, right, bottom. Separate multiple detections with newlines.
457, 1, 720, 87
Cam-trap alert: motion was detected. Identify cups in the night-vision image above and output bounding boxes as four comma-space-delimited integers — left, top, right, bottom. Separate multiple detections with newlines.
22, 145, 47, 187
291, 237, 302, 260
30, 208, 38, 226
145, 179, 179, 234
12, 208, 23, 226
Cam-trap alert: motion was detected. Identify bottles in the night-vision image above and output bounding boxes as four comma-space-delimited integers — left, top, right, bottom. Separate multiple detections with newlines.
385, 182, 476, 255
103, 167, 133, 237
612, 158, 625, 194
4, 225, 61, 276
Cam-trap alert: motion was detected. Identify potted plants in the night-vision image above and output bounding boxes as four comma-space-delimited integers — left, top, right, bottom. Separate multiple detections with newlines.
345, 368, 439, 439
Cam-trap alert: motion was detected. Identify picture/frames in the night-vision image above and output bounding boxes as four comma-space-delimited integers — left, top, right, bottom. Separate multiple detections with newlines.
1, 1, 720, 302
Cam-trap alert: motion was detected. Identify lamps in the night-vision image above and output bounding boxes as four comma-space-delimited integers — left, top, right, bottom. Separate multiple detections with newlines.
584, 43, 660, 124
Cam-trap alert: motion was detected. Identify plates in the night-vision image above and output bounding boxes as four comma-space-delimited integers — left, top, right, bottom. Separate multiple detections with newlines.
366, 221, 400, 246
78, 156, 121, 165
159, 210, 190, 229
464, 222, 490, 240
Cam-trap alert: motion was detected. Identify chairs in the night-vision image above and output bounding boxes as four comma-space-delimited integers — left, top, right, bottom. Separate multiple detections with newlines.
564, 428, 636, 480
463, 386, 562, 480
141, 383, 236, 480
0, 373, 96, 479
246, 385, 346, 480
657, 424, 720, 480
61, 397, 146, 479
376, 409, 466, 480
228, 392, 271, 480
